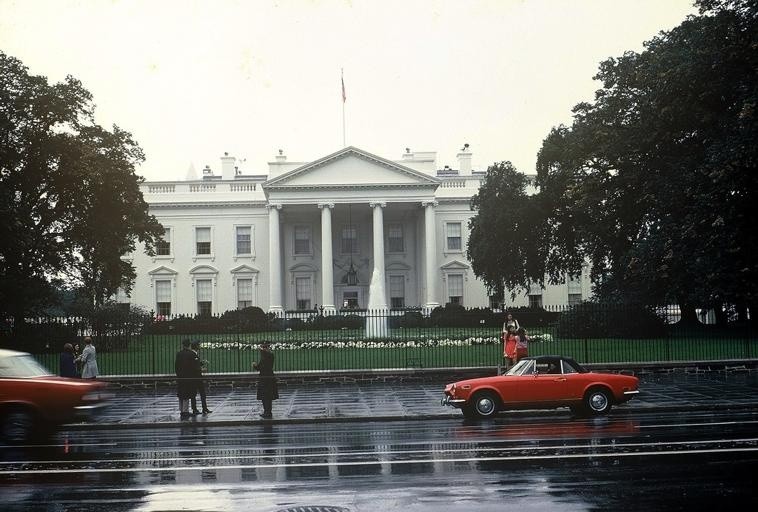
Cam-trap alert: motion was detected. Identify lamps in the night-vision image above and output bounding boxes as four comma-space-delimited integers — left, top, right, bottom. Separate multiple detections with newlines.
346, 204, 357, 286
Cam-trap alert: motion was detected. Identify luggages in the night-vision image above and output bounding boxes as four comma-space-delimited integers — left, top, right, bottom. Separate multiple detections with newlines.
497, 356, 507, 375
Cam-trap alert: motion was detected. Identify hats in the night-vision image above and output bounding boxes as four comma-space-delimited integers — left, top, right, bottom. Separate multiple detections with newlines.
182, 338, 202, 347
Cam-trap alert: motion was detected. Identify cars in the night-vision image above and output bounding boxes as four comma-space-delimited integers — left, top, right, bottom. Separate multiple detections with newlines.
0, 348, 113, 448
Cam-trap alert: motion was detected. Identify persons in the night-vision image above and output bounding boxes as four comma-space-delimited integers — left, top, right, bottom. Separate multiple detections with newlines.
513, 328, 530, 361
71, 341, 81, 377
173, 338, 201, 417
58, 342, 76, 378
503, 325, 516, 369
190, 339, 213, 414
73, 335, 98, 379
250, 341, 280, 420
502, 313, 520, 369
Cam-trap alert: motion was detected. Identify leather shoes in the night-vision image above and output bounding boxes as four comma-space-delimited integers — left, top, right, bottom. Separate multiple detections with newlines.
181, 409, 213, 417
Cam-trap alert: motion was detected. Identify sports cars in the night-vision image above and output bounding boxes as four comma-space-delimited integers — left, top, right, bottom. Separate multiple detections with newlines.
440, 355, 641, 416
